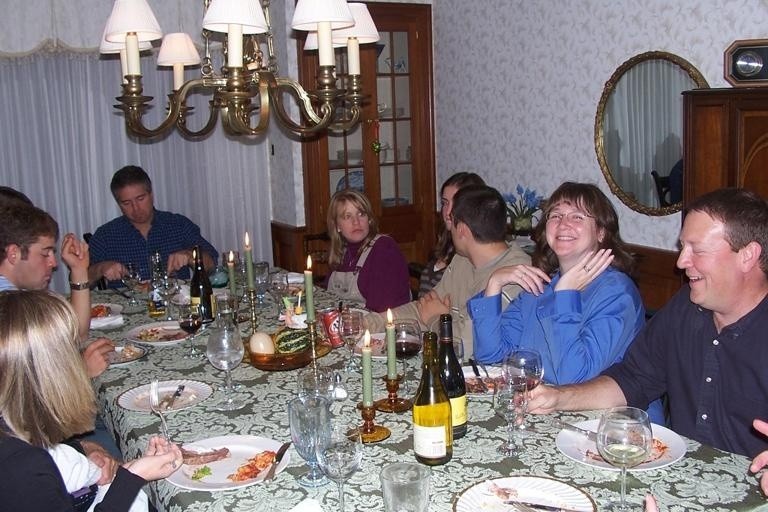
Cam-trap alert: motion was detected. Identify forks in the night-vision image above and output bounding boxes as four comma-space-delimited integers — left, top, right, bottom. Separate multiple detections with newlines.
149, 379, 176, 469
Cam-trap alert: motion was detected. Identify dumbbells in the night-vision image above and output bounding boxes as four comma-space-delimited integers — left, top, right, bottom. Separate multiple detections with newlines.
273, 327, 317, 354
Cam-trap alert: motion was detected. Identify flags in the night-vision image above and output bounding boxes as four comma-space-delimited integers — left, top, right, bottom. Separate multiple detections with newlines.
512, 216, 531, 233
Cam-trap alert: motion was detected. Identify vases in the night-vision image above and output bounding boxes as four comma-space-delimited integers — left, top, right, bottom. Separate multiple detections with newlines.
503, 184, 544, 216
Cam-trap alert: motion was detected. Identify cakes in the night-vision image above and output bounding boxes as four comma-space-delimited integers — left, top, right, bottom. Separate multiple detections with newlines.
320, 307, 346, 348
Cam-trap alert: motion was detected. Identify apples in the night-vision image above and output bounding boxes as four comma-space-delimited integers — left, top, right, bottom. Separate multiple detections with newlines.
385, 308, 397, 381
244, 231, 255, 290
362, 330, 373, 406
304, 256, 315, 321
227, 251, 236, 294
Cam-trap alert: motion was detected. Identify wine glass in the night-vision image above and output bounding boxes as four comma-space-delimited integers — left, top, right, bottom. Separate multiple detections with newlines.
83, 275, 465, 348
495, 390, 526, 455
596, 406, 652, 510
583, 266, 591, 273
462, 362, 523, 400
501, 349, 544, 432
207, 349, 247, 410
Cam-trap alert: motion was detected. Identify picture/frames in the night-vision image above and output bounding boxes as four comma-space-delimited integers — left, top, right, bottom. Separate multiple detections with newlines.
626, 237, 689, 319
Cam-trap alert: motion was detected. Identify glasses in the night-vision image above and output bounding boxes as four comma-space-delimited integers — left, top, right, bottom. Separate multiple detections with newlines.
99, 0, 380, 140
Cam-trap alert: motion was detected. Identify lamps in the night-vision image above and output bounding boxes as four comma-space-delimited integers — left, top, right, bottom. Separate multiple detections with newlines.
651, 170, 672, 208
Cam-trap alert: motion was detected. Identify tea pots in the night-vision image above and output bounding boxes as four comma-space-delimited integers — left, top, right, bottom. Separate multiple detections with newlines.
593, 51, 711, 216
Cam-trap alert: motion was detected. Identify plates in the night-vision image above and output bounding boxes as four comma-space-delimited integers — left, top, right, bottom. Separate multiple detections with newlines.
249, 349, 310, 372
165, 435, 288, 492
452, 476, 599, 511
555, 419, 685, 473
681, 87, 768, 225
118, 378, 212, 413
295, 0, 437, 299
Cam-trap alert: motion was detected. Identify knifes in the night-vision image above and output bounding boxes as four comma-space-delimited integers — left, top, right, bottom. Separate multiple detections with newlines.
554, 422, 621, 446
261, 442, 292, 484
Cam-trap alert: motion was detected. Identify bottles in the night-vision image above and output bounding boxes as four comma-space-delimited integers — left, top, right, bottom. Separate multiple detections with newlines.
413, 348, 454, 465
440, 350, 469, 439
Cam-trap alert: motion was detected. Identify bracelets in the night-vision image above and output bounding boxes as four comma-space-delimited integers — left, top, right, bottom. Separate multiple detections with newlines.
69, 279, 90, 290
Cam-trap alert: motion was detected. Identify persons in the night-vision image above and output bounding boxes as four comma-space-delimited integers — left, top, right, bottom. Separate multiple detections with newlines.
514, 187, 768, 460
2, 186, 123, 461
418, 172, 486, 300
465, 181, 666, 426
645, 419, 768, 512
669, 158, 684, 206
326, 189, 413, 313
88, 166, 218, 289
0, 289, 184, 512
359, 183, 533, 365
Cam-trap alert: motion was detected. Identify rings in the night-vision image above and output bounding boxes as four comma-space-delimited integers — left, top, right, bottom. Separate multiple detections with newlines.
583, 266, 591, 273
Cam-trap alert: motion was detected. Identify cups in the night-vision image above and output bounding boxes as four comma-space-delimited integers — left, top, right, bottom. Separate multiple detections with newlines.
297, 366, 337, 402
316, 423, 362, 510
289, 395, 329, 487
544, 210, 602, 223
379, 461, 431, 509
